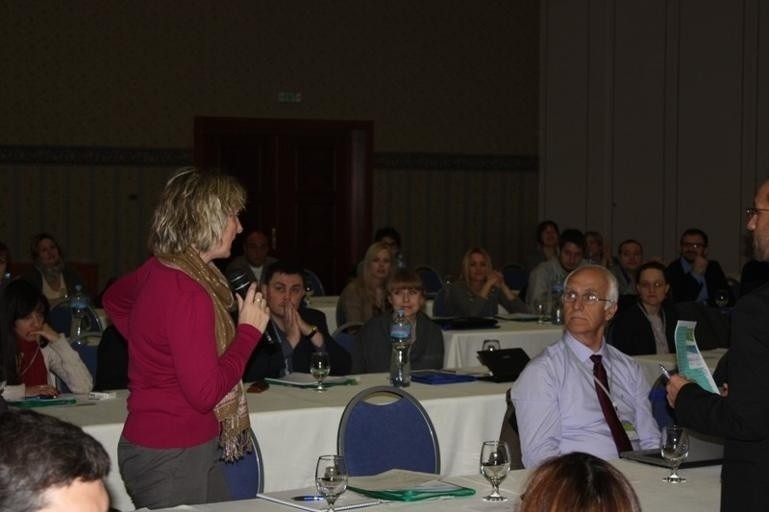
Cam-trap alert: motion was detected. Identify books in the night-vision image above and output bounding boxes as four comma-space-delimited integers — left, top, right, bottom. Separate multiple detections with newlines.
256, 481, 394, 512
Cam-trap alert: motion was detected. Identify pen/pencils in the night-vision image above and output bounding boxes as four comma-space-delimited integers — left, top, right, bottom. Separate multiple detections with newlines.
441, 369, 456, 372
659, 365, 671, 380
291, 494, 325, 501
76, 403, 96, 406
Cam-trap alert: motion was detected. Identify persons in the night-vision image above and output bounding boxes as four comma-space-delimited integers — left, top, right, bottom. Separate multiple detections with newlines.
510, 264, 663, 472
0, 409, 111, 511
518, 451, 640, 512
0, 233, 130, 405
101, 164, 272, 511
665, 179, 767, 512
223, 219, 769, 384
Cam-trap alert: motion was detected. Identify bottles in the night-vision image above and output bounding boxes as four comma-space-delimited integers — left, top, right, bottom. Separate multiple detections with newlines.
69, 284, 89, 349
387, 308, 414, 388
551, 275, 565, 326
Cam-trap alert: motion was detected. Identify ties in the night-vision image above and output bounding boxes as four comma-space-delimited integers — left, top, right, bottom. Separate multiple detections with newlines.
586, 355, 633, 457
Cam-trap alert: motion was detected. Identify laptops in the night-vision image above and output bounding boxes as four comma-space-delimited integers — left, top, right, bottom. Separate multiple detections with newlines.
470, 348, 530, 383
620, 387, 723, 470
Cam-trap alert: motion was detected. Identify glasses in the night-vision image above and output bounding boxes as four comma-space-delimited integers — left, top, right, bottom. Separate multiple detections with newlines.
742, 207, 769, 225
682, 242, 704, 248
561, 293, 614, 303
637, 282, 665, 289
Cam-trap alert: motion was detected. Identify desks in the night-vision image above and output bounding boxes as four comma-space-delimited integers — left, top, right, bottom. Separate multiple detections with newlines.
2, 365, 516, 511
429, 317, 565, 371
135, 455, 722, 512
625, 346, 729, 398
301, 294, 340, 336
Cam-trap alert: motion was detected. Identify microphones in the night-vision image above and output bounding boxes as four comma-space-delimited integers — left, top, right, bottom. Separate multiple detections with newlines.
225, 263, 276, 345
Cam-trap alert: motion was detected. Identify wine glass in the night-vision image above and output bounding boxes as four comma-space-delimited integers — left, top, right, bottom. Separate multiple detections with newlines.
307, 350, 332, 393
715, 287, 729, 309
658, 422, 691, 484
303, 282, 314, 306
479, 338, 501, 353
532, 297, 547, 324
477, 440, 514, 504
312, 454, 351, 512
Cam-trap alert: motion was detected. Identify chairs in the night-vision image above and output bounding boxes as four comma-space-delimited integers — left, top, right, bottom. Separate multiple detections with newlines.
331, 320, 367, 358
336, 385, 441, 480
498, 260, 528, 297
648, 369, 690, 431
432, 285, 451, 317
55, 332, 106, 393
410, 263, 446, 299
219, 426, 264, 500
40, 297, 104, 346
302, 269, 325, 296
